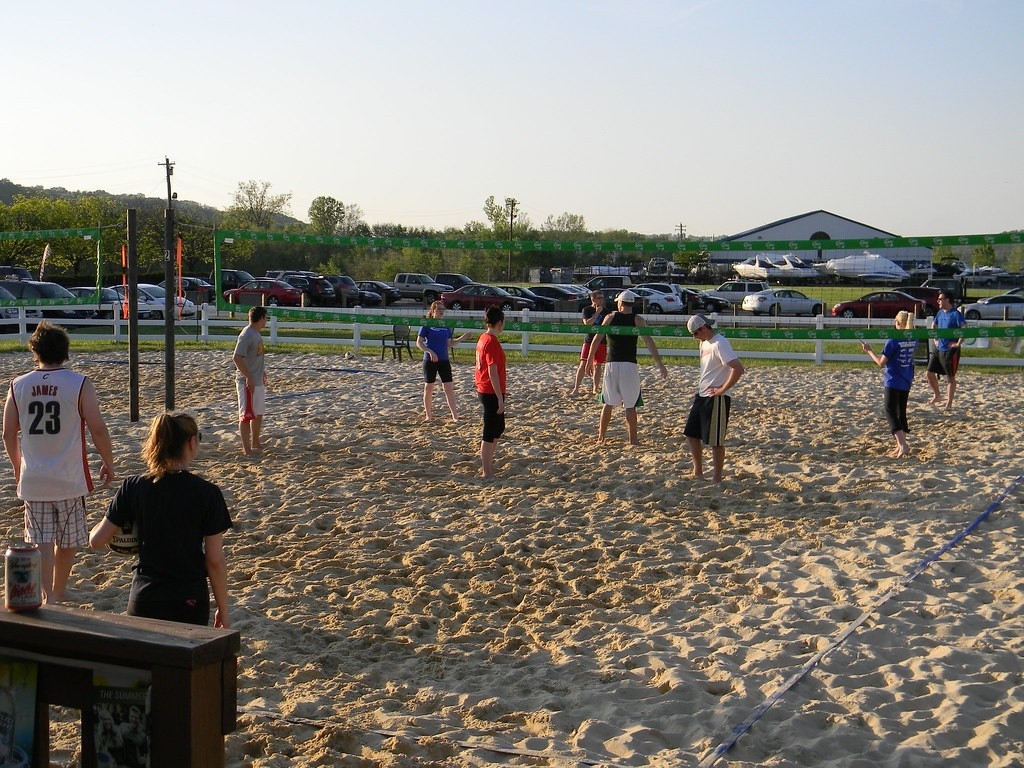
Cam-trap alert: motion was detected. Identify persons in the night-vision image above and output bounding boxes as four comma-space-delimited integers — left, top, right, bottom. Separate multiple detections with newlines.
416, 301, 474, 422
89, 410, 233, 628
859, 310, 920, 459
683, 313, 744, 483
570, 290, 607, 395
232, 306, 267, 455
584, 290, 668, 445
476, 306, 506, 478
2, 318, 114, 605
926, 292, 968, 411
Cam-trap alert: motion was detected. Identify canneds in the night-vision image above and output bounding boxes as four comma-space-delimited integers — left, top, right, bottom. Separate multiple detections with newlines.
4, 542, 42, 611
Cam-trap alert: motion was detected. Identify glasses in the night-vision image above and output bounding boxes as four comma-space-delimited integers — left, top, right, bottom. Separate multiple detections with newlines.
594, 297, 605, 300
691, 329, 700, 337
937, 297, 948, 301
434, 306, 446, 310
189, 433, 203, 442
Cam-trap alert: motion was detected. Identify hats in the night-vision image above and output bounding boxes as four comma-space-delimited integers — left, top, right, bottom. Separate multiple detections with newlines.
614, 291, 635, 303
687, 313, 715, 332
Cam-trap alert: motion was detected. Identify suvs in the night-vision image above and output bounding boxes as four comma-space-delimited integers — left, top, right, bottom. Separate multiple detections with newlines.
266, 270, 336, 306
701, 281, 771, 303
323, 276, 359, 306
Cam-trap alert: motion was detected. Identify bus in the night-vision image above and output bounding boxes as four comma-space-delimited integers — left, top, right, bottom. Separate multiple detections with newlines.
623, 259, 690, 277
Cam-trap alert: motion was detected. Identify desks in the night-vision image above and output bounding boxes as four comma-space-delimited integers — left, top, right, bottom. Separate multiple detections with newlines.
0, 597, 240, 768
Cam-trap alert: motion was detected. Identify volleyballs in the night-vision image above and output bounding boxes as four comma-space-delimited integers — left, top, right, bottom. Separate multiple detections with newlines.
105, 520, 142, 557
344, 351, 355, 360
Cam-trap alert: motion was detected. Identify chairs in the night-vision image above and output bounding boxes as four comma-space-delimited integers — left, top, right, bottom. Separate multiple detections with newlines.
912, 339, 940, 380
381, 324, 412, 363
449, 327, 455, 360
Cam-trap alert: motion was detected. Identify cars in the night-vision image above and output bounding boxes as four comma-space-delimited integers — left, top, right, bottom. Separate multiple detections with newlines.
0, 280, 87, 326
157, 277, 215, 303
0, 286, 43, 330
222, 277, 303, 306
55, 287, 152, 319
481, 286, 560, 311
741, 288, 825, 316
831, 271, 1024, 321
440, 285, 536, 311
529, 285, 732, 313
361, 291, 381, 307
111, 283, 195, 319
355, 281, 401, 306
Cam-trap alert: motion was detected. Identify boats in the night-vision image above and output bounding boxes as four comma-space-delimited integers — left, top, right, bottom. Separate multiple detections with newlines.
733, 252, 1007, 279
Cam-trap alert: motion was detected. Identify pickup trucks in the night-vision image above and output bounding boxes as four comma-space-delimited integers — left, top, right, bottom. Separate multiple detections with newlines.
584, 276, 682, 291
415, 273, 472, 301
197, 268, 259, 289
363, 273, 454, 303
